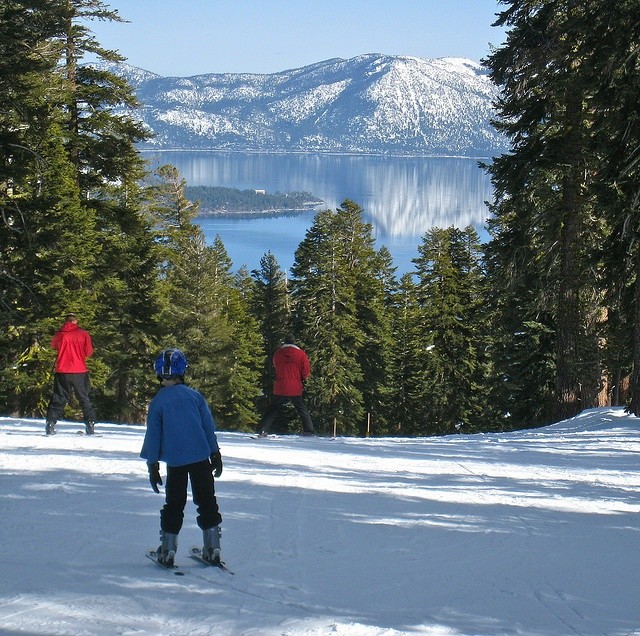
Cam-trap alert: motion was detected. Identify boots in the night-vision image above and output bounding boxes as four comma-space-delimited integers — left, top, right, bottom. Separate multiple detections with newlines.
201, 526, 221, 566
45, 419, 57, 436
85, 421, 95, 435
156, 531, 177, 569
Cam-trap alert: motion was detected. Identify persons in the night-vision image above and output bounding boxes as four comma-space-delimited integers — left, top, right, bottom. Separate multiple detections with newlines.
140, 349, 222, 568
46, 314, 96, 435
259, 334, 315, 437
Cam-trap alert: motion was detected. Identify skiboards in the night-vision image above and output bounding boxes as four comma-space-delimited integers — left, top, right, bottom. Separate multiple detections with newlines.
146, 546, 236, 575
43, 431, 103, 437
249, 434, 336, 441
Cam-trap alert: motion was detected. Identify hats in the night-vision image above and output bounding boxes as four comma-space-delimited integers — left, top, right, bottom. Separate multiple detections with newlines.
284, 333, 295, 344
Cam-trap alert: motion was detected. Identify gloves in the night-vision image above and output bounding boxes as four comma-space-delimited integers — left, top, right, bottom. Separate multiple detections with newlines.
146, 462, 163, 494
209, 450, 223, 478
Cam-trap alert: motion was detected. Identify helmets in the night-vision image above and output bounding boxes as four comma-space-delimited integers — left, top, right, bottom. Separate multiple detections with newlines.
154, 350, 188, 383
